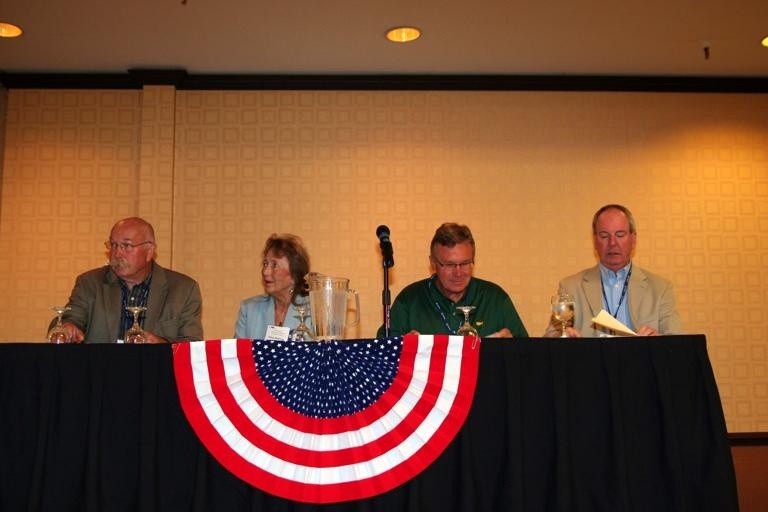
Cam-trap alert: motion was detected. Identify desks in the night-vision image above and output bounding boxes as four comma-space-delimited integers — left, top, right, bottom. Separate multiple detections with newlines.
0, 333, 741, 511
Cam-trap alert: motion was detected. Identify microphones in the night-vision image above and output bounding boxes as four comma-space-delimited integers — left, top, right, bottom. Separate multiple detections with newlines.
375, 225, 395, 268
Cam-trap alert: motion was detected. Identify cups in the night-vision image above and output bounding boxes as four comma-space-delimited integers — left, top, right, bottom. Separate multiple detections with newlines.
306, 276, 361, 339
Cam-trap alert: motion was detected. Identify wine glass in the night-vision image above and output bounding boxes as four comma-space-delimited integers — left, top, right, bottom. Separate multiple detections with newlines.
455, 306, 479, 336
291, 305, 314, 341
552, 293, 575, 338
124, 306, 149, 344
44, 305, 71, 342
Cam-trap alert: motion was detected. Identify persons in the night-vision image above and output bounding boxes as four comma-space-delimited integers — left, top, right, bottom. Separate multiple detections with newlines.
376, 222, 530, 338
544, 204, 684, 337
48, 216, 202, 344
232, 233, 338, 340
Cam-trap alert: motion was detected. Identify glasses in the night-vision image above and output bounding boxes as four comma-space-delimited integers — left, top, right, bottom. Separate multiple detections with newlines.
433, 255, 474, 271
104, 241, 151, 252
